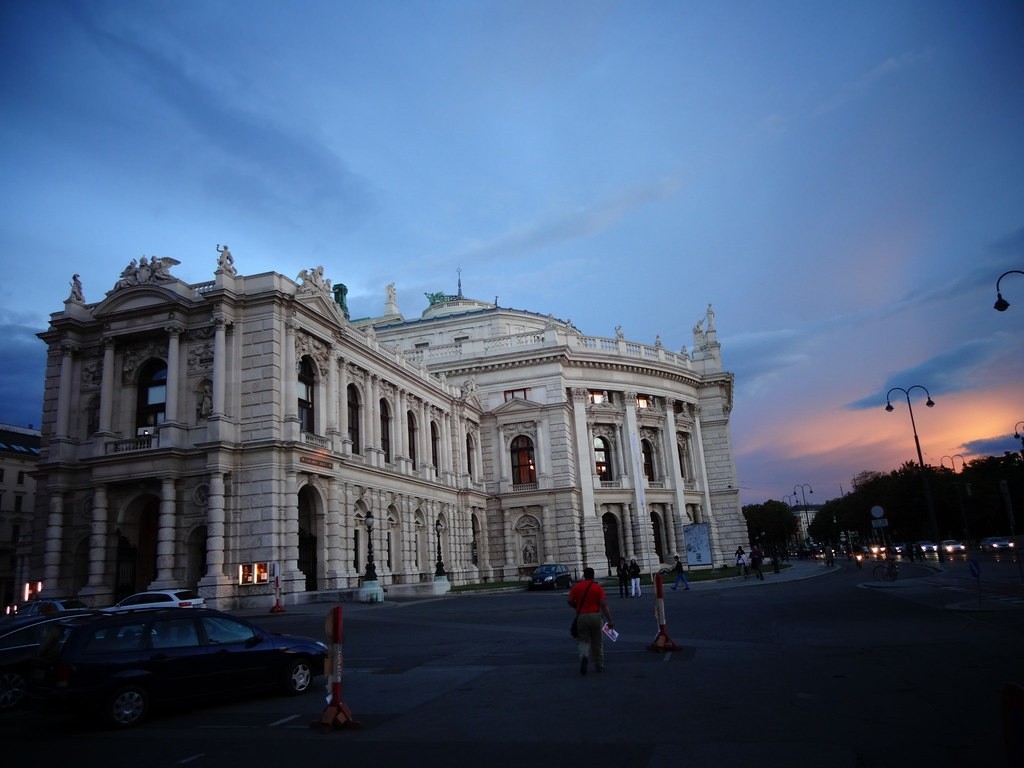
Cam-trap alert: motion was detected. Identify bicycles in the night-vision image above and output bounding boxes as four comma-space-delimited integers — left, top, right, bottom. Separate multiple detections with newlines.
872, 557, 903, 581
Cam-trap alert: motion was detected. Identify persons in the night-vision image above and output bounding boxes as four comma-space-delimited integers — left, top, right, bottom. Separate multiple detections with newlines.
119, 254, 181, 278
617, 556, 643, 599
826, 549, 834, 568
567, 568, 615, 675
749, 546, 765, 581
460, 374, 479, 397
201, 385, 213, 420
424, 289, 446, 304
317, 265, 339, 305
735, 546, 746, 581
523, 539, 536, 565
615, 325, 624, 339
386, 282, 396, 302
655, 335, 662, 347
905, 542, 926, 562
693, 303, 715, 333
69, 274, 86, 303
669, 556, 690, 591
216, 243, 237, 275
680, 345, 687, 355
882, 548, 896, 581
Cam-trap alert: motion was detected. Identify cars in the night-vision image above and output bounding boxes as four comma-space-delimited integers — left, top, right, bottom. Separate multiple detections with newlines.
11, 600, 87, 621
918, 540, 937, 552
939, 539, 966, 555
528, 563, 572, 591
845, 543, 917, 560
0, 607, 114, 729
979, 537, 1015, 555
98, 590, 208, 615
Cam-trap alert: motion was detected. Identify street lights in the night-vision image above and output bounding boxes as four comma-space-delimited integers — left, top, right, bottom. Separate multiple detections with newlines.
791, 483, 814, 527
434, 520, 448, 577
937, 454, 966, 476
780, 495, 799, 511
364, 510, 379, 580
885, 385, 944, 562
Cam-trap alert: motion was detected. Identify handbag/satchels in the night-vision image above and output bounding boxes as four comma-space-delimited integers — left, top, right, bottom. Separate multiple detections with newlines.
570, 623, 578, 638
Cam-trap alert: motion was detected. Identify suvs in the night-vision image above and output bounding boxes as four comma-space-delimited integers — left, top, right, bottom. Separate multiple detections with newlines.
27, 605, 328, 729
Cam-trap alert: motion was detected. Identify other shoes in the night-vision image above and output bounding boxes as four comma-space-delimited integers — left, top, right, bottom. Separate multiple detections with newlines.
594, 664, 606, 671
684, 588, 690, 590
581, 655, 588, 675
671, 587, 676, 590
